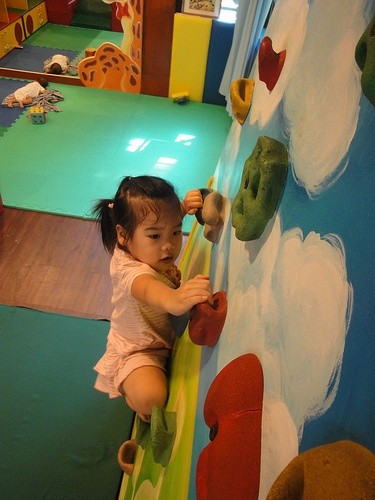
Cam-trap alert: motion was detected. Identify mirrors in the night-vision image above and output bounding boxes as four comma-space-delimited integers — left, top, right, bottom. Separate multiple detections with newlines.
0, 0, 143, 95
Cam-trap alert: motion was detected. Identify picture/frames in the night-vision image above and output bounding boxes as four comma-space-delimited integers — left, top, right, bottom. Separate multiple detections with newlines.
182, 0, 221, 19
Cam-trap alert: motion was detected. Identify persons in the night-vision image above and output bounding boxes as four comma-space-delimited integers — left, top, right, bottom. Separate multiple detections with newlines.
7, 77, 48, 109
42, 54, 79, 74
88, 174, 215, 421
